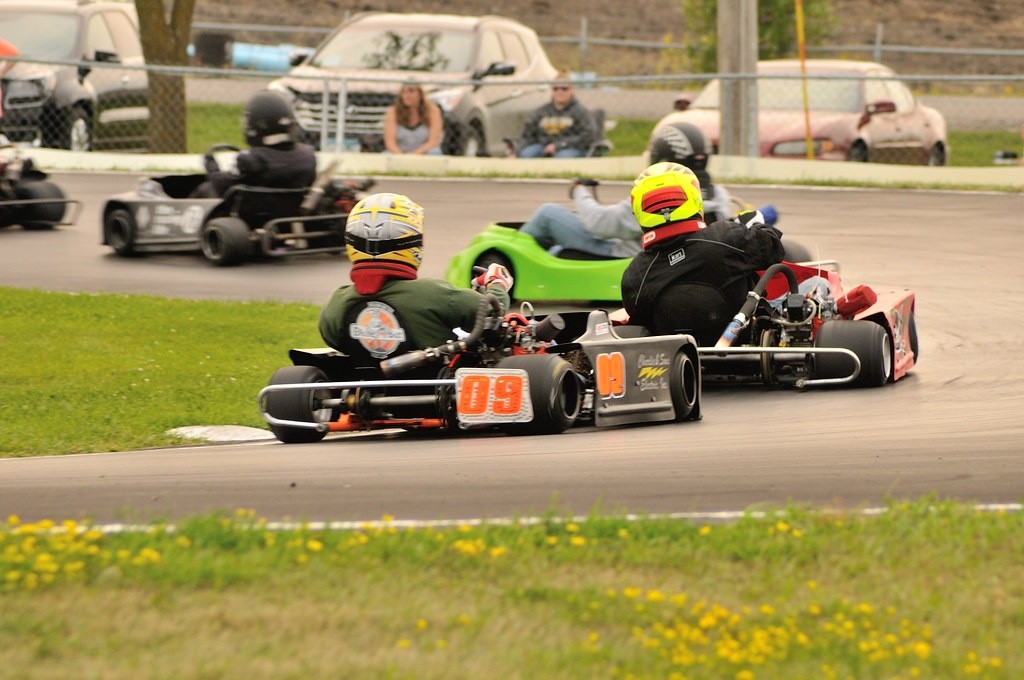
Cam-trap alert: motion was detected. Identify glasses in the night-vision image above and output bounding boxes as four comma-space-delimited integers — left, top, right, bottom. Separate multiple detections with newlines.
552, 87, 568, 92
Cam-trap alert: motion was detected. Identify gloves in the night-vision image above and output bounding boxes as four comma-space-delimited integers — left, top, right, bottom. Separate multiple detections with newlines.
471, 263, 513, 293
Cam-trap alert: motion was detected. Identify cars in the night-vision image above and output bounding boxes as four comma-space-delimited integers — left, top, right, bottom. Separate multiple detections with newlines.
642, 58, 949, 169
261, 10, 568, 158
2, 2, 153, 150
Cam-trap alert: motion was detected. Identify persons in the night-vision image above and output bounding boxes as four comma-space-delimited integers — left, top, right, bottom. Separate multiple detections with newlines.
189, 89, 316, 200
621, 162, 829, 344
376, 81, 443, 155
516, 119, 728, 259
514, 73, 597, 156
318, 192, 513, 359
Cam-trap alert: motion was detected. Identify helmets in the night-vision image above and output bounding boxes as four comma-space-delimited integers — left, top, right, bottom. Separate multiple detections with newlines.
629, 162, 707, 249
649, 122, 709, 171
243, 90, 297, 151
345, 192, 424, 294
